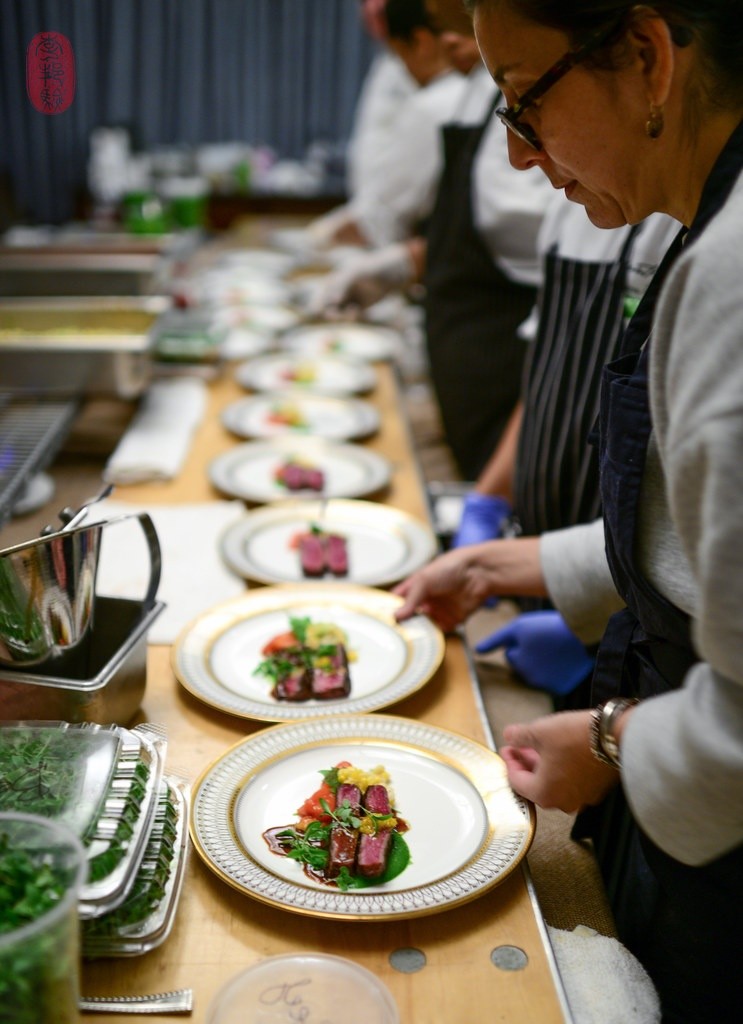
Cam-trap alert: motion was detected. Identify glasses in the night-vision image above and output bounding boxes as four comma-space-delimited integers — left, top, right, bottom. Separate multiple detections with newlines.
495, 14, 694, 152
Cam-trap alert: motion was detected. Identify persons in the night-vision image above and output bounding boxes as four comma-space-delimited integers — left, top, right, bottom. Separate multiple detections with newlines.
455, 179, 689, 696
391, 1, 743, 1021
279, 1, 578, 492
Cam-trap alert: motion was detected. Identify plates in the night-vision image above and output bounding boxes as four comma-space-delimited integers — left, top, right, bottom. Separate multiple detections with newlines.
218, 497, 439, 586
170, 580, 446, 723
213, 322, 397, 442
188, 712, 540, 923
207, 440, 394, 500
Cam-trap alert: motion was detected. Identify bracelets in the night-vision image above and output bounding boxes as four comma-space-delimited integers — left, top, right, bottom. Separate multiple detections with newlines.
588, 687, 639, 773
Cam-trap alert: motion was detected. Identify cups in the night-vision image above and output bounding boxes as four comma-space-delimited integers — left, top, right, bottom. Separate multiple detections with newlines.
0, 810, 87, 1024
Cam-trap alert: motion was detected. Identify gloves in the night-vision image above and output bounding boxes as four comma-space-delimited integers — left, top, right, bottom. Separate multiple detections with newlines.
307, 245, 415, 321
453, 493, 510, 607
475, 610, 596, 697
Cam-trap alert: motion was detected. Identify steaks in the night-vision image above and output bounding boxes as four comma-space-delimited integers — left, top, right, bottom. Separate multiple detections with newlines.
276, 651, 307, 698
330, 782, 361, 867
300, 535, 349, 577
358, 785, 391, 875
284, 465, 323, 490
312, 644, 351, 699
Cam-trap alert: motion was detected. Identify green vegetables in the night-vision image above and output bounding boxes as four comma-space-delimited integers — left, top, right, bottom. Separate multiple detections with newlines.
253, 617, 336, 686
0, 558, 51, 661
276, 479, 289, 486
312, 527, 321, 534
0, 727, 178, 1024
275, 767, 394, 889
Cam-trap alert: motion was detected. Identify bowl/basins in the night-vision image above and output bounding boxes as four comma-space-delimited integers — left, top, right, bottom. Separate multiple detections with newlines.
0, 510, 193, 955
